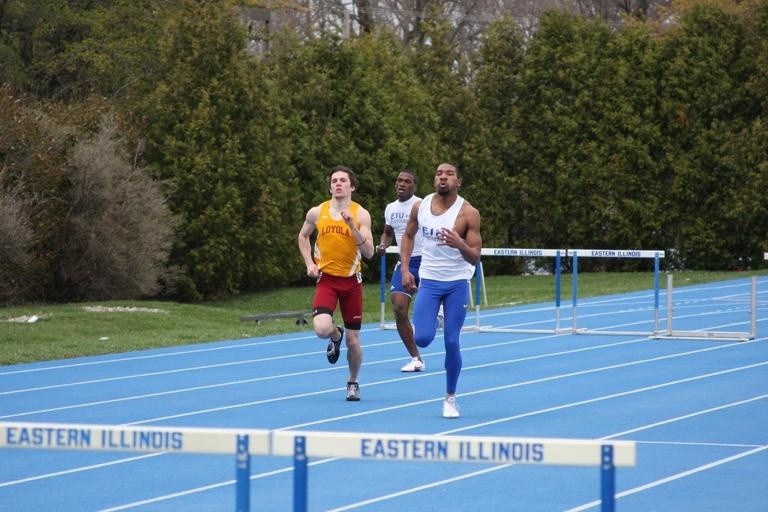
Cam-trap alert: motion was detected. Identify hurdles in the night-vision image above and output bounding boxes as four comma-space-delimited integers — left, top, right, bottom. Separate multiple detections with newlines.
380, 245, 493, 334
1, 421, 272, 512
648, 273, 758, 341
273, 429, 638, 511
473, 246, 572, 335
564, 249, 668, 336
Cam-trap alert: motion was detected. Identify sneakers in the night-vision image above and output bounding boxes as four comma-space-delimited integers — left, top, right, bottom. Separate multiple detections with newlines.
442, 397, 460, 418
400, 356, 425, 372
438, 304, 444, 318
346, 380, 361, 401
326, 325, 345, 364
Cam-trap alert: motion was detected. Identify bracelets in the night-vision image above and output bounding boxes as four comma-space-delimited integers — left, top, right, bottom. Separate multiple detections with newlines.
355, 238, 366, 247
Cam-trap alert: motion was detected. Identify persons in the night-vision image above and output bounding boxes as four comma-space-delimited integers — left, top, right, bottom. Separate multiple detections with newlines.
297, 164, 375, 401
377, 169, 426, 373
400, 160, 483, 420
